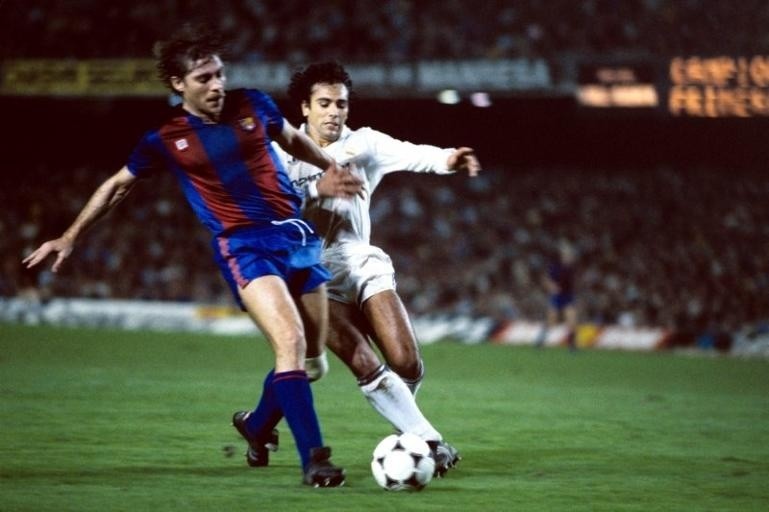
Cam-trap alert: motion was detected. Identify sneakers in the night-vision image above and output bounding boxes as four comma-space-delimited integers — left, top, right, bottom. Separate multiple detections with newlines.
303, 446, 346, 489
427, 438, 459, 478
232, 411, 280, 468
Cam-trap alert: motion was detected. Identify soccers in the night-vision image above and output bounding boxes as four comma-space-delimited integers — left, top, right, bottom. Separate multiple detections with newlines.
371, 432, 435, 492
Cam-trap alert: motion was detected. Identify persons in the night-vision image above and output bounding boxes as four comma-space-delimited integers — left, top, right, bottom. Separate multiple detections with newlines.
269, 55, 485, 479
22, 25, 372, 488
1, 2, 769, 357
